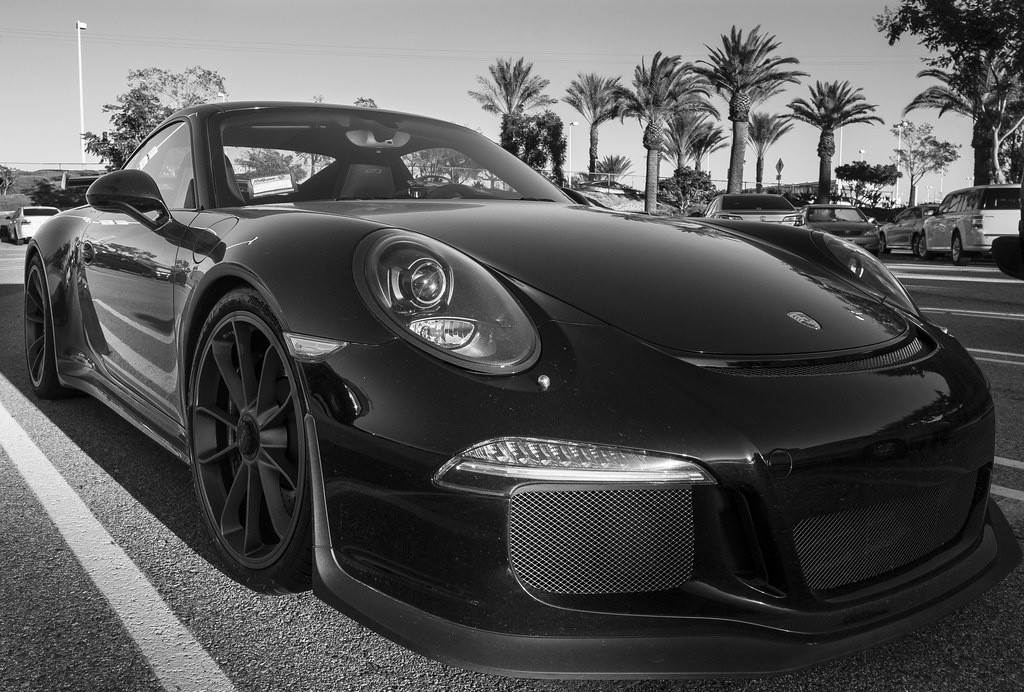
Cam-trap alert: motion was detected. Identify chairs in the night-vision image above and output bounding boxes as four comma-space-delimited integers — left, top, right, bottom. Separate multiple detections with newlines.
175, 153, 396, 203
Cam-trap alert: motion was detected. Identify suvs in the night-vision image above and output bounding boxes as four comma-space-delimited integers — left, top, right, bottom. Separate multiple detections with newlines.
918, 184, 1022, 266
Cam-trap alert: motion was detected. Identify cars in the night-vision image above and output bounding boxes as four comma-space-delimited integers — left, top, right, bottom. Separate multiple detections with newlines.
56, 169, 100, 190
877, 203, 940, 258
5, 206, 63, 246
690, 194, 800, 227
576, 179, 643, 200
798, 204, 881, 258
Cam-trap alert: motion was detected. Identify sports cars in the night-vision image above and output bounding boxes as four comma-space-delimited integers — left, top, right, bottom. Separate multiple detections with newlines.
24, 102, 1024, 680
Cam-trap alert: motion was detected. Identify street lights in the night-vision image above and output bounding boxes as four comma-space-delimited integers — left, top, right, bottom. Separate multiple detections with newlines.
896, 121, 908, 205
941, 156, 950, 202
568, 121, 579, 188
75, 20, 88, 163
858, 149, 864, 162
